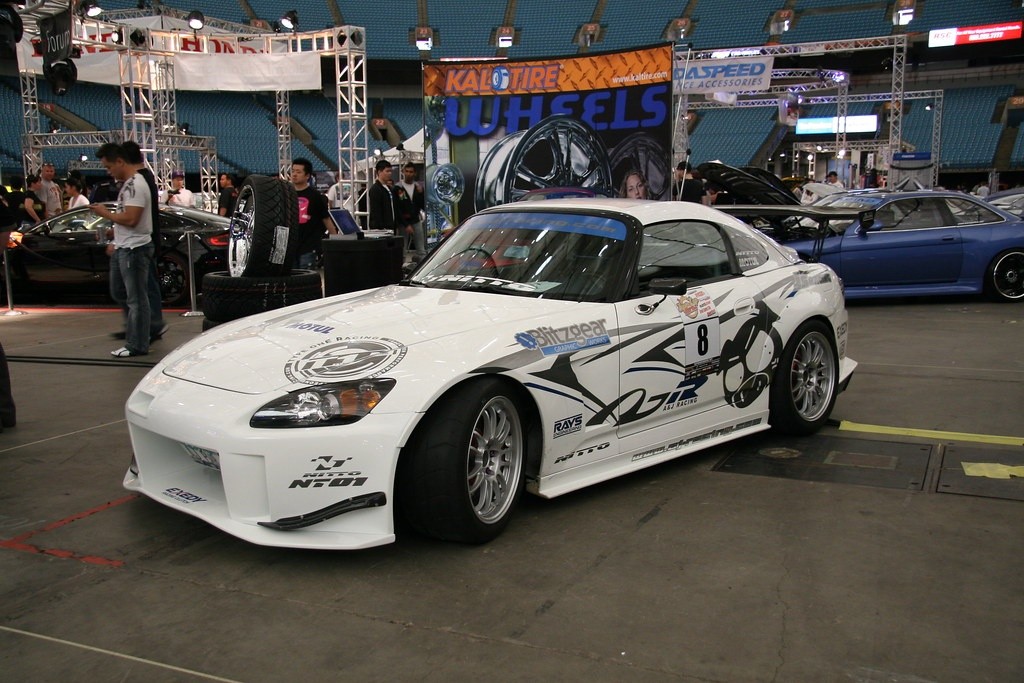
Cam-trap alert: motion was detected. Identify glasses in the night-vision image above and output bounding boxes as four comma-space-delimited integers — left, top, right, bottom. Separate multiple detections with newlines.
42, 162, 54, 168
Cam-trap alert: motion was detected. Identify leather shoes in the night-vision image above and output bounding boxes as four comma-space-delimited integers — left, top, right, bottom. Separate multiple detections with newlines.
109, 331, 126, 340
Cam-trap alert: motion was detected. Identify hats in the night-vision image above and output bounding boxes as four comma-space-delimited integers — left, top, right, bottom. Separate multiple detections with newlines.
172, 171, 185, 180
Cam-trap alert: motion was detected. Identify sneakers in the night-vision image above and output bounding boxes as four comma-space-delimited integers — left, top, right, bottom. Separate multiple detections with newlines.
110, 346, 130, 357
149, 323, 169, 340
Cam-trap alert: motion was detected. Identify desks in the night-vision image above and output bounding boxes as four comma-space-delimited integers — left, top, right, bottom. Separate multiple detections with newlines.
320, 236, 409, 296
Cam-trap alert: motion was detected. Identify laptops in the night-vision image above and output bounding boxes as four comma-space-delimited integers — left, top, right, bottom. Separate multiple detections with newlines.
327, 209, 392, 239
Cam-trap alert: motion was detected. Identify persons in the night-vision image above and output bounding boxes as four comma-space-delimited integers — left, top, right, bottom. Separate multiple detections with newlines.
218, 173, 238, 217
0, 161, 94, 227
358, 160, 398, 237
977, 181, 990, 197
291, 158, 337, 270
162, 169, 196, 209
824, 171, 843, 189
672, 161, 716, 206
87, 141, 170, 357
619, 168, 652, 200
328, 171, 356, 218
397, 162, 427, 265
800, 178, 818, 205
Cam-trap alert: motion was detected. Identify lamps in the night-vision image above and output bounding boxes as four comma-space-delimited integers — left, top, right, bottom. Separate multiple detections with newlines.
412, 24, 435, 51
47, 120, 58, 134
398, 144, 406, 153
925, 102, 935, 111
186, 9, 204, 30
374, 148, 382, 157
764, 9, 798, 36
662, 18, 691, 44
176, 122, 191, 134
78, 153, 88, 162
281, 10, 300, 32
495, 26, 517, 49
109, 28, 121, 44
578, 23, 598, 47
84, 2, 102, 18
885, 1, 918, 26
130, 28, 146, 47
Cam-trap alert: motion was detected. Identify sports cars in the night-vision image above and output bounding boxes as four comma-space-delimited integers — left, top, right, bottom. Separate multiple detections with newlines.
738, 167, 913, 230
952, 187, 1024, 222
121, 197, 859, 551
0, 200, 236, 307
695, 160, 1024, 303
